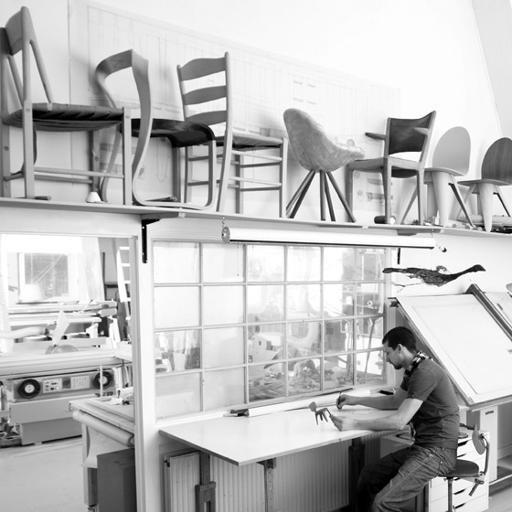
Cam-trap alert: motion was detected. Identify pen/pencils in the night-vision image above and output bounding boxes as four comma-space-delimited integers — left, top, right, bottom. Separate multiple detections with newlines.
338, 392, 342, 405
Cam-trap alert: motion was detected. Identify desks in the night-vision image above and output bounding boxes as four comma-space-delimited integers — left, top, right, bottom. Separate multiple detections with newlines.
65, 395, 132, 508
154, 398, 417, 511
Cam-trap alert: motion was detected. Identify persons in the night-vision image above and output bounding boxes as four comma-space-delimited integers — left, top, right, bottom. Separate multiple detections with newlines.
248, 316, 273, 350
331, 327, 460, 512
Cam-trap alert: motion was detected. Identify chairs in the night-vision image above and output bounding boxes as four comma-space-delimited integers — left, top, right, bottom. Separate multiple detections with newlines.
177, 50, 285, 220
94, 49, 215, 209
284, 106, 364, 222
400, 126, 476, 230
1, 6, 131, 206
344, 110, 437, 226
436, 422, 489, 511
454, 136, 511, 232
